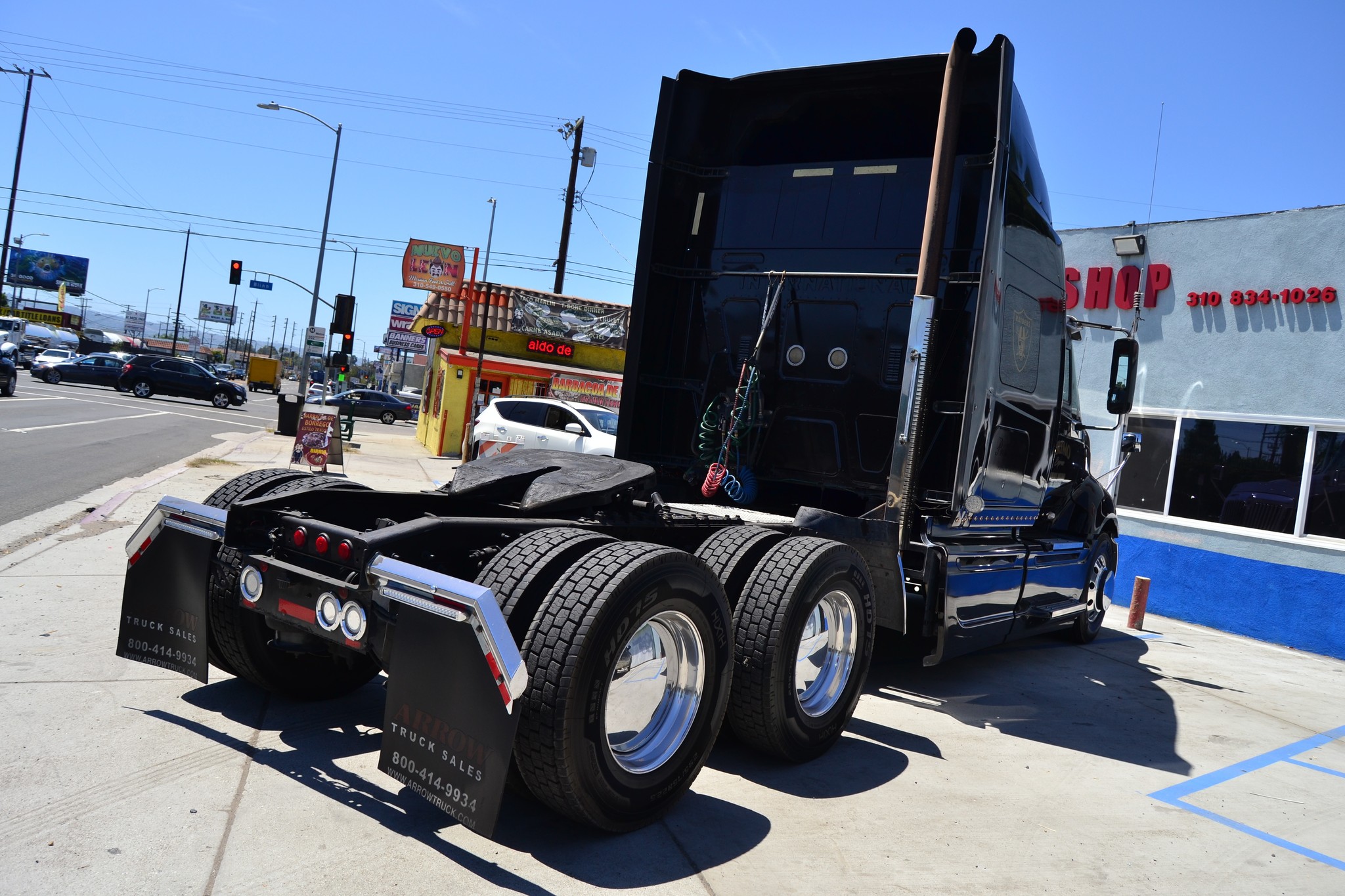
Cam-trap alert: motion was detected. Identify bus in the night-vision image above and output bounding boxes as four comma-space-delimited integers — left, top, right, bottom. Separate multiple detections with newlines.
308, 369, 325, 387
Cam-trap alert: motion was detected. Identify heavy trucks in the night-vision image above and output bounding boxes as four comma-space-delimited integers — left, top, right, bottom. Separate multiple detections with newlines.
115, 27, 1141, 845
0, 314, 80, 370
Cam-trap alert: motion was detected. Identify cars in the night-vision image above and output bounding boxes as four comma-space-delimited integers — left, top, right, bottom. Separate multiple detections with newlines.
0, 349, 17, 397
75, 351, 137, 367
31, 349, 78, 367
305, 383, 333, 398
349, 383, 368, 391
28, 354, 132, 392
397, 388, 422, 399
288, 375, 298, 381
209, 362, 246, 381
305, 388, 414, 424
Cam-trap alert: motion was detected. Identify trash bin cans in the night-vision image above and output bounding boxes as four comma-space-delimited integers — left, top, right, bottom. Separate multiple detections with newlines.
277, 391, 304, 435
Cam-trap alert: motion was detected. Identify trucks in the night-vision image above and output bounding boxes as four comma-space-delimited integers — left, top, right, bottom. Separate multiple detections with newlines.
247, 356, 284, 395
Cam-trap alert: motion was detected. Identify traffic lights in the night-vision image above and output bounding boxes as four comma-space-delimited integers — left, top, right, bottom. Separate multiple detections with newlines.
365, 376, 367, 379
229, 259, 242, 286
341, 332, 354, 354
340, 365, 349, 371
332, 353, 350, 366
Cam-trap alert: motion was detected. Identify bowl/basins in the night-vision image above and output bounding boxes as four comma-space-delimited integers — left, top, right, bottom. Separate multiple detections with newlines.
306, 451, 328, 467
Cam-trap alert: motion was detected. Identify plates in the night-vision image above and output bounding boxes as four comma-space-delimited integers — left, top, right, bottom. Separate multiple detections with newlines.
593, 322, 625, 338
572, 333, 591, 342
561, 308, 598, 325
536, 315, 571, 334
525, 301, 550, 317
302, 432, 329, 448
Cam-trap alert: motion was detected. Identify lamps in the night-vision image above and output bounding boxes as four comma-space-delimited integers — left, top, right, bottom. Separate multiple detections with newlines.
1112, 234, 1145, 256
457, 369, 463, 379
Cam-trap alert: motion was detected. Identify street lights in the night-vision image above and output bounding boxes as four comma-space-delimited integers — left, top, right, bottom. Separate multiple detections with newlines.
326, 239, 358, 296
254, 101, 342, 397
359, 349, 367, 359
240, 330, 255, 367
355, 339, 366, 383
11, 233, 50, 316
142, 288, 165, 340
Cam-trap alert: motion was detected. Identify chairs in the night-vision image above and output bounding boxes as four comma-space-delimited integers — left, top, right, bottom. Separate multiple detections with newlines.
548, 413, 561, 429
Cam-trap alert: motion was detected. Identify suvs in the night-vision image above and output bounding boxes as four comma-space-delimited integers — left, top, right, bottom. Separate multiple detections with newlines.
175, 354, 226, 380
472, 394, 619, 461
118, 353, 248, 409
